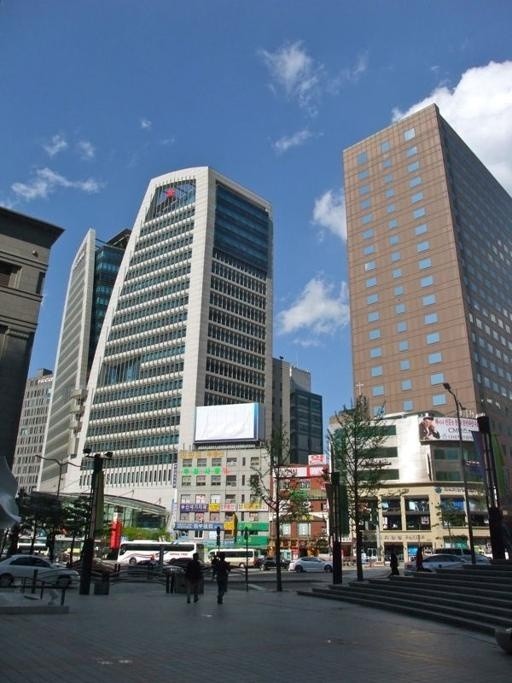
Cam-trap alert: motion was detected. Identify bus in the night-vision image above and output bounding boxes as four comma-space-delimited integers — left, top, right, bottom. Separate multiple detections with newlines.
117, 540, 262, 576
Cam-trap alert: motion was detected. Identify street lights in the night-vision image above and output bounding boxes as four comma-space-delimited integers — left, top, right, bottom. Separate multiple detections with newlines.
35, 454, 87, 495
442, 381, 476, 565
80, 448, 113, 595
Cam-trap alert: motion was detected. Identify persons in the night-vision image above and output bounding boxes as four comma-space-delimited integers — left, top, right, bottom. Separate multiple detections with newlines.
185, 553, 201, 603
419, 417, 441, 441
388, 553, 400, 578
416, 547, 424, 571
211, 555, 219, 577
212, 553, 231, 604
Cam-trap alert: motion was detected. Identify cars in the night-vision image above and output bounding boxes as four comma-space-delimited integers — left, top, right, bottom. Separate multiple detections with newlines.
255, 556, 333, 573
404, 548, 493, 577
0, 554, 121, 588
